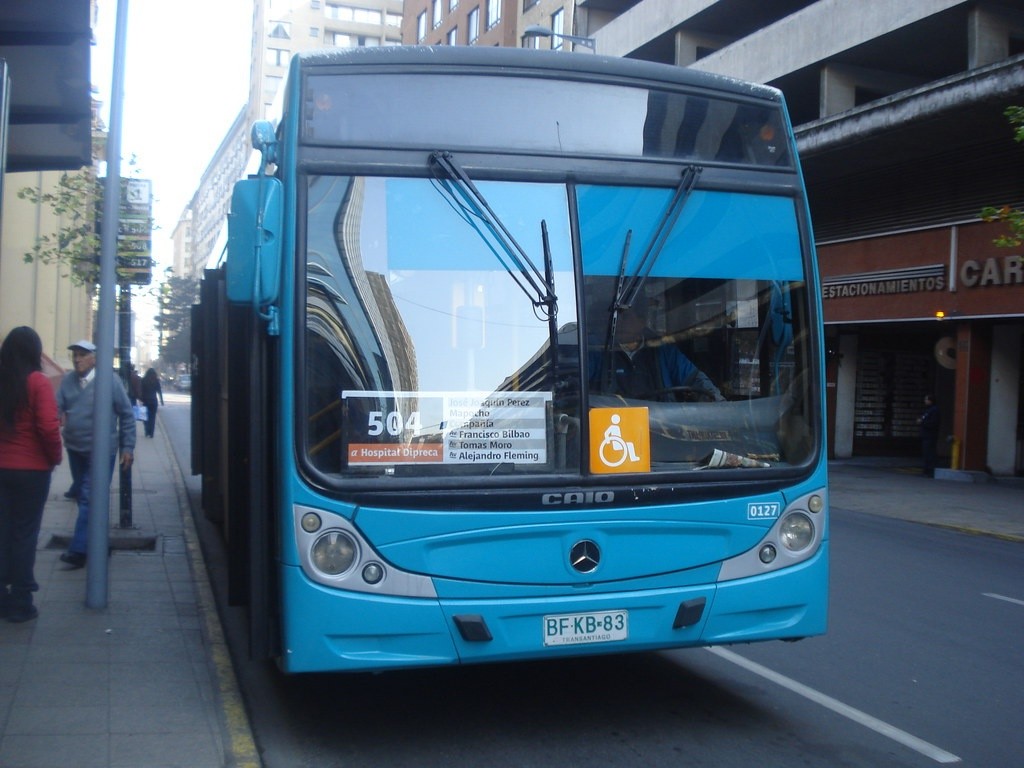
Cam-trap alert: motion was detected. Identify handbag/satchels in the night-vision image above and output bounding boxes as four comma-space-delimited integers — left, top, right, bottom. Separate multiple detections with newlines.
133, 403, 147, 422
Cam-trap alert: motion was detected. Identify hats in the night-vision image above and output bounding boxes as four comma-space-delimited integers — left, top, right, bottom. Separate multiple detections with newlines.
67, 340, 97, 351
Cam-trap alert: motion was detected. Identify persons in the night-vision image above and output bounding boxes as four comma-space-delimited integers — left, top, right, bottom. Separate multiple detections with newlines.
915, 394, 943, 478
55, 339, 165, 561
0, 325, 64, 623
587, 292, 725, 404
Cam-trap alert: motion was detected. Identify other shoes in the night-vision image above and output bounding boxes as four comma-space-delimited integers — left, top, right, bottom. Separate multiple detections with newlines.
0, 588, 38, 622
63, 488, 77, 498
59, 549, 87, 567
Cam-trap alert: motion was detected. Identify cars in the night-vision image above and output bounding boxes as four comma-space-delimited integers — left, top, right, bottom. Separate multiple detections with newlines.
173, 374, 191, 392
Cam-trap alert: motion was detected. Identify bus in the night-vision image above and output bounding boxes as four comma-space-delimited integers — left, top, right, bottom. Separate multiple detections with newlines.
189, 42, 834, 679
